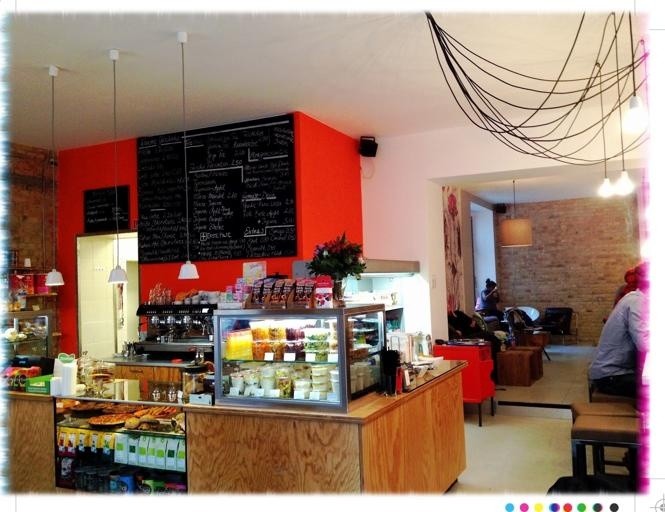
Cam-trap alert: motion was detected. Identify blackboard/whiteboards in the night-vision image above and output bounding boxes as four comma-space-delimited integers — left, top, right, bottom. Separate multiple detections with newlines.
137, 112, 297, 264
83, 185, 130, 233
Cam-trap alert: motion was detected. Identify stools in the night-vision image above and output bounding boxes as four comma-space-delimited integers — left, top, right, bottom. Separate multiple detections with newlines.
571, 388, 641, 481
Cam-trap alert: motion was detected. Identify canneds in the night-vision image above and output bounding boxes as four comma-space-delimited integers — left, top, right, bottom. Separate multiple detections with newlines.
147, 381, 183, 402
74, 464, 109, 492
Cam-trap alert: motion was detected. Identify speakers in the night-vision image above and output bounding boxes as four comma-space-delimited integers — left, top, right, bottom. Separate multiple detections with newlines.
359, 135, 379, 158
496, 204, 506, 214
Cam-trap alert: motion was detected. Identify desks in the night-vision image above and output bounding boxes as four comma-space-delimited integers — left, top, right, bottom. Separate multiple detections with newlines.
497, 346, 543, 386
434, 341, 495, 426
517, 331, 551, 361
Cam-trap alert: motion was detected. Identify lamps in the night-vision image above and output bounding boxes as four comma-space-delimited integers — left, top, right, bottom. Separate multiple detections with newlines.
500, 178, 533, 249
106, 48, 127, 284
422, 9, 648, 196
176, 31, 200, 281
45, 64, 65, 287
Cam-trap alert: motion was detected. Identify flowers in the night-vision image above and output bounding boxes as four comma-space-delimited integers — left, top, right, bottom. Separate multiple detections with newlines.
305, 232, 368, 281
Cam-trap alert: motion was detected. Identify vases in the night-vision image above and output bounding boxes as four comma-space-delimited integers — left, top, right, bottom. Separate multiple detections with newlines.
332, 274, 347, 308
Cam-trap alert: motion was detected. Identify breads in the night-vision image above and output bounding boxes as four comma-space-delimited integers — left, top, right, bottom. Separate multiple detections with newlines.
87, 406, 177, 431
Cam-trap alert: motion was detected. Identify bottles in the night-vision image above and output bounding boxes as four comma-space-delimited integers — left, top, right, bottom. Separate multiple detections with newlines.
3, 317, 33, 341
113, 364, 215, 407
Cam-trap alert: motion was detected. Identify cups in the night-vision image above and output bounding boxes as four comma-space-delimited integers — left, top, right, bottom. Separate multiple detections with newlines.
8, 275, 48, 312
379, 365, 428, 396
223, 318, 374, 401
149, 287, 247, 309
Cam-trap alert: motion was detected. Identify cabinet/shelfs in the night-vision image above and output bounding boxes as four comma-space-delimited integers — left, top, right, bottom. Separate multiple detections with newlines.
55, 397, 186, 498
4, 268, 62, 358
115, 364, 182, 384
214, 304, 387, 413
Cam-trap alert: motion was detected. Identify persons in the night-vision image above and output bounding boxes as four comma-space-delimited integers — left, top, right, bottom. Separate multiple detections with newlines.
586, 281, 648, 397
474, 279, 503, 320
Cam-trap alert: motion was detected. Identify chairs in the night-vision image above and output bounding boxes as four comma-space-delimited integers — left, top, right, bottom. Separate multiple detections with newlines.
536, 308, 578, 346
